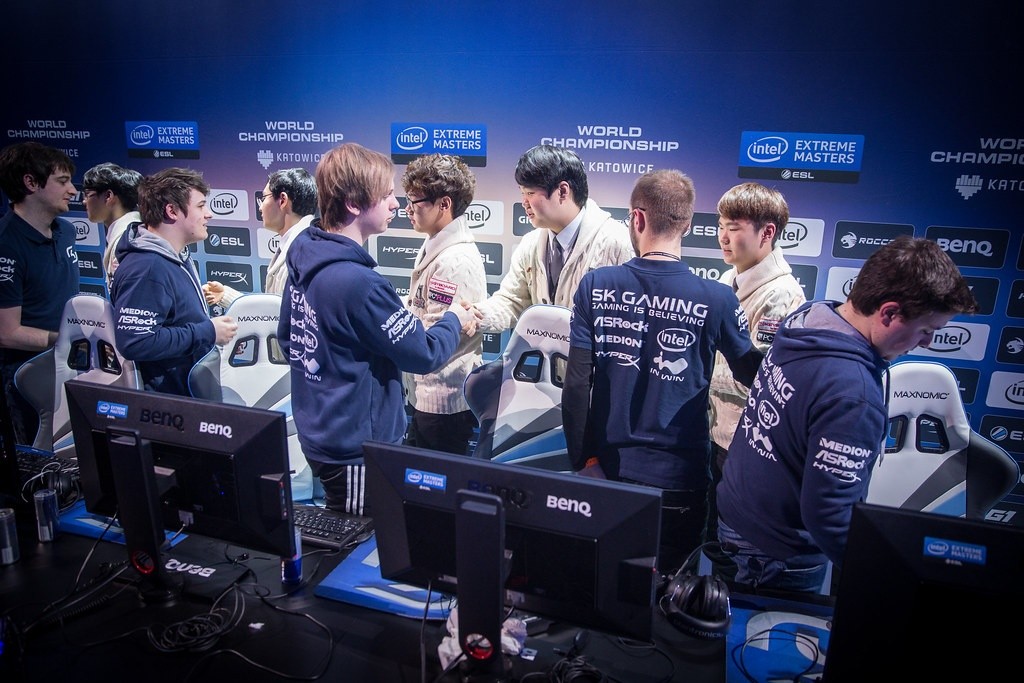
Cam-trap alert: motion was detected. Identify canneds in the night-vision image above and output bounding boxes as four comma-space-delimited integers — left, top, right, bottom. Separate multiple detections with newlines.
279, 526, 302, 584
0, 508, 20, 565
33, 489, 60, 542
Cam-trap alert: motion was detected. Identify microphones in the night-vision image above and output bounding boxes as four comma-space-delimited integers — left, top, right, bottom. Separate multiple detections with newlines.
668, 542, 740, 581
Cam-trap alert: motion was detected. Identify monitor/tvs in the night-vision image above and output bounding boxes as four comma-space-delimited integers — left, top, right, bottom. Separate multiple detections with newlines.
0, 386, 20, 496
64, 379, 297, 605
361, 440, 663, 683
820, 502, 1024, 683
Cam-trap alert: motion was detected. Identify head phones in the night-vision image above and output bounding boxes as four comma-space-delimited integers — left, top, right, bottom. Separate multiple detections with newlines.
664, 575, 733, 639
22, 469, 80, 514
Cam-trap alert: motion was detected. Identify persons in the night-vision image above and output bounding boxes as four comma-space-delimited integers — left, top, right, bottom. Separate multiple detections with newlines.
82, 162, 145, 298
400, 154, 487, 453
202, 168, 319, 364
109, 167, 238, 398
706, 181, 808, 475
460, 143, 637, 339
276, 142, 484, 520
716, 235, 977, 599
0, 141, 80, 443
562, 169, 766, 573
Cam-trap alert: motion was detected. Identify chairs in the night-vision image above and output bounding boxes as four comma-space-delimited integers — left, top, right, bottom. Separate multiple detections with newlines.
465, 304, 596, 476
859, 360, 1020, 523
188, 293, 326, 503
14, 293, 145, 461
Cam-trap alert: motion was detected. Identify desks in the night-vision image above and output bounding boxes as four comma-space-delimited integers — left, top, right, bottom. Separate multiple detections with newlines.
0, 443, 838, 683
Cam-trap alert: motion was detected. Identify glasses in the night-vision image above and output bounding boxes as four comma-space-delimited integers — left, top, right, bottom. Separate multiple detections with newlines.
82, 188, 95, 199
256, 193, 272, 207
622, 206, 646, 228
407, 195, 429, 212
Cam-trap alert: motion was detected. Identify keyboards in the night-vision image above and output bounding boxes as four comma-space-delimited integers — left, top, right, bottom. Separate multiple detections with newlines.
15, 444, 80, 481
292, 505, 372, 551
